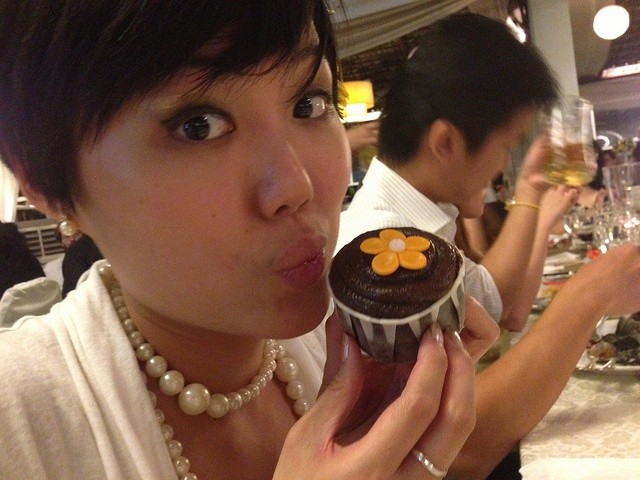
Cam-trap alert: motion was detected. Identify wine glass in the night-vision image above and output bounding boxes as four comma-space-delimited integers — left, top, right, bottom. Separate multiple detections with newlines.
550, 96, 598, 234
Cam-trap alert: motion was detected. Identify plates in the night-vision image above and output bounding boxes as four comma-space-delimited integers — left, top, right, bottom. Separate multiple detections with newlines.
575, 319, 639, 377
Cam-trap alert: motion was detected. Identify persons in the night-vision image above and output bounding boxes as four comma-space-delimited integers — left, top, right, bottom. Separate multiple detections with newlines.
564, 138, 617, 206
2, 0, 502, 478
453, 131, 578, 333
56, 227, 104, 299
311, 8, 640, 479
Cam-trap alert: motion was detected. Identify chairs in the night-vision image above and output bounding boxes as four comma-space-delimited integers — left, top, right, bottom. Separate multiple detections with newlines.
0, 276, 62, 331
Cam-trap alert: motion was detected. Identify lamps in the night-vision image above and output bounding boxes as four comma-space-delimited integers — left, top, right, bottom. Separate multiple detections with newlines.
336, 79, 382, 125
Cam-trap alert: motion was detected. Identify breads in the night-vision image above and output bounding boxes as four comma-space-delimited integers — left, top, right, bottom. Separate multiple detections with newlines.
328, 226, 466, 365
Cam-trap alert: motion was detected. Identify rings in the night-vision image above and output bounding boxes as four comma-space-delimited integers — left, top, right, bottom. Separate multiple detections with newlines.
410, 447, 448, 478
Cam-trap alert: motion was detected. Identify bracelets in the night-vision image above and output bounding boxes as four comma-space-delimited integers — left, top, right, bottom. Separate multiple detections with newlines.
505, 199, 541, 214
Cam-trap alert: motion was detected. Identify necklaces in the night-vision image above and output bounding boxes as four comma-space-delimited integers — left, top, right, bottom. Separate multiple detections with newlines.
98, 265, 313, 480
